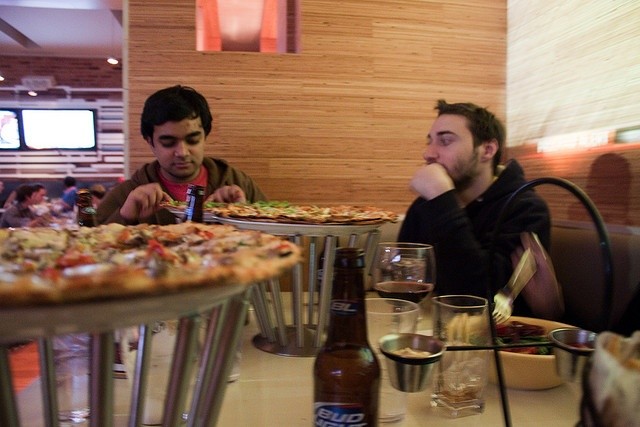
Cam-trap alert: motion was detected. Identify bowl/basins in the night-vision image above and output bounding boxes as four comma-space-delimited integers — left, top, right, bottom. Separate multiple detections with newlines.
379, 332, 445, 392
547, 328, 602, 380
446, 311, 575, 391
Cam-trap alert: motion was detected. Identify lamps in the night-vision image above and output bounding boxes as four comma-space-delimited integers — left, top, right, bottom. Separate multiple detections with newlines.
106, 11, 120, 65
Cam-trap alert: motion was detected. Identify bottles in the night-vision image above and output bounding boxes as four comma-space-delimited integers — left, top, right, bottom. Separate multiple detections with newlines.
311, 246, 380, 425
184, 185, 205, 222
74, 190, 96, 228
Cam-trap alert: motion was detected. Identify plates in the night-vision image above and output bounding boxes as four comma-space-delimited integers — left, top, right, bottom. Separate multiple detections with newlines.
170, 210, 213, 221
0, 282, 250, 345
212, 213, 380, 237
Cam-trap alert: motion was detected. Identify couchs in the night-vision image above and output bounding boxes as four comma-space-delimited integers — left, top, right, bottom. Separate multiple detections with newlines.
547, 219, 639, 338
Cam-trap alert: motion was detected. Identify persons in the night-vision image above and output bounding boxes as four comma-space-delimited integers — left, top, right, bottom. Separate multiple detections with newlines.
92, 84, 269, 226
510, 231, 639, 338
397, 98, 551, 316
0, 177, 105, 231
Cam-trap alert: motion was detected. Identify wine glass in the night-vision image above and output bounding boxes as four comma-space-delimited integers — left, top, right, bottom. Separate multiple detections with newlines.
371, 242, 438, 332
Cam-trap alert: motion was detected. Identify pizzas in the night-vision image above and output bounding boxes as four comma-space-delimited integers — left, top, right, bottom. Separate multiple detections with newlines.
159, 199, 399, 225
1, 220, 304, 305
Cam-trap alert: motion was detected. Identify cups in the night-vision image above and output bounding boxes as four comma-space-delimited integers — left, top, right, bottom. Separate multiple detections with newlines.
38, 332, 91, 425
362, 295, 420, 422
429, 293, 489, 414
